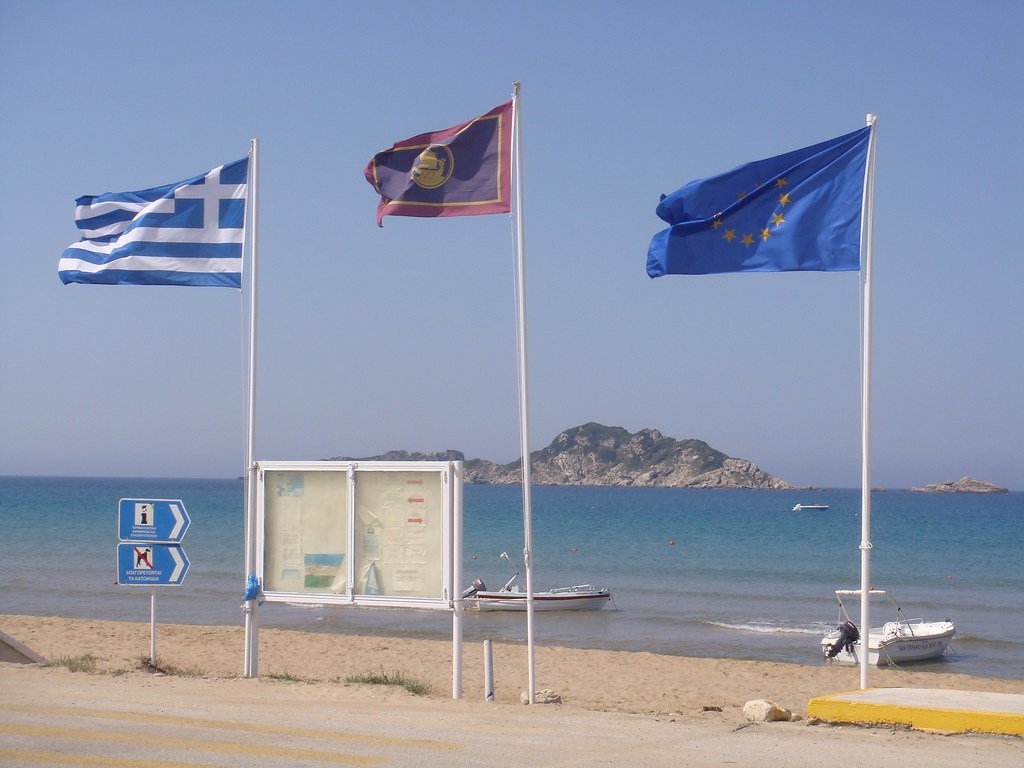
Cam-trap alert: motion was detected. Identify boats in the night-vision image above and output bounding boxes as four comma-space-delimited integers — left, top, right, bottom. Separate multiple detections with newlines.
458, 551, 613, 613
791, 503, 830, 511
820, 586, 957, 668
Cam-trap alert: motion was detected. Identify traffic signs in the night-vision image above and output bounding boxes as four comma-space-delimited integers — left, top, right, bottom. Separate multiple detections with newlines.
116, 541, 192, 587
117, 497, 192, 544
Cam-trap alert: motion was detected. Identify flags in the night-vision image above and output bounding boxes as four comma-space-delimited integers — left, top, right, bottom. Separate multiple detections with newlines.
646, 120, 874, 279
57, 154, 251, 291
364, 99, 515, 228
